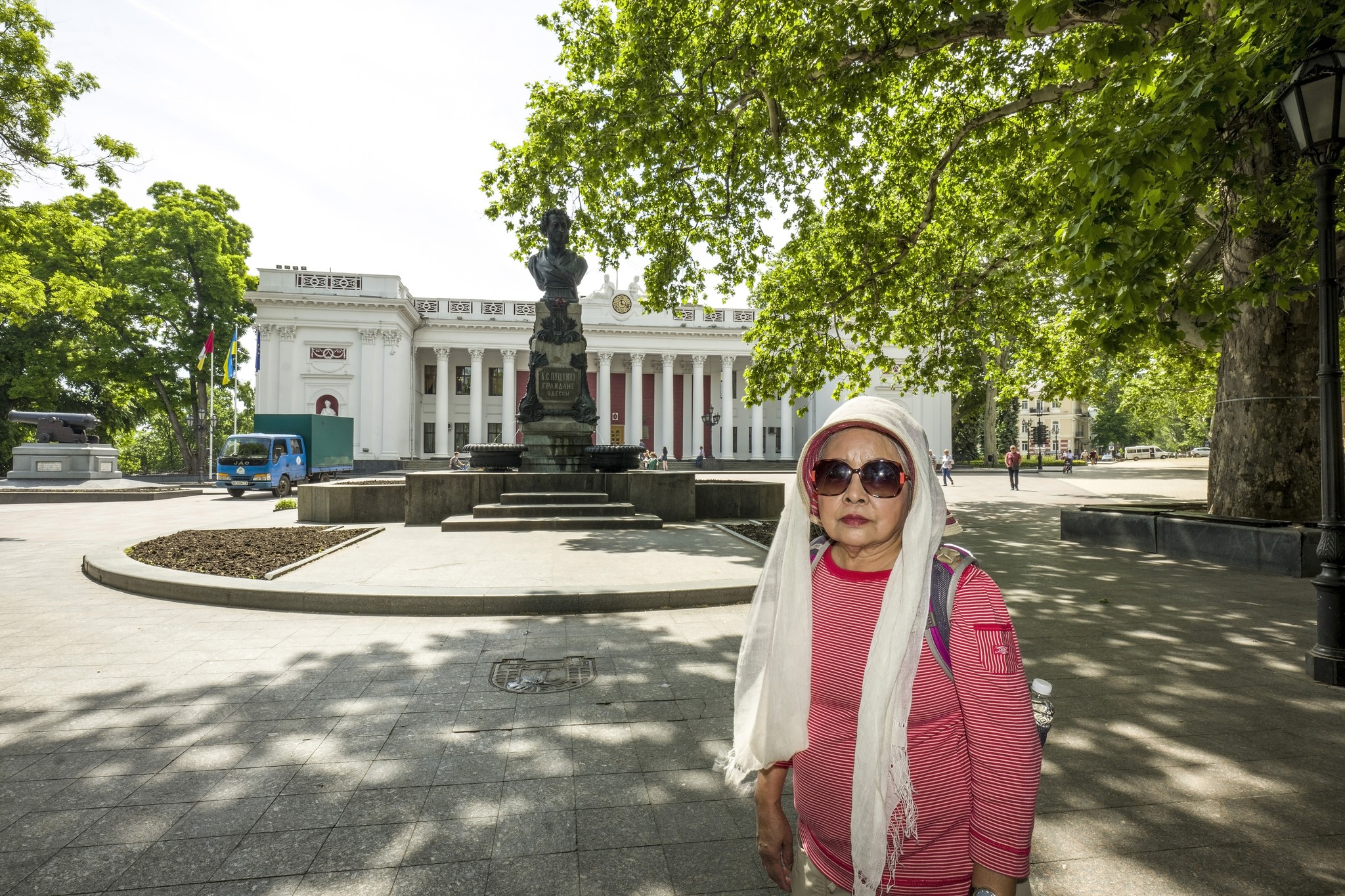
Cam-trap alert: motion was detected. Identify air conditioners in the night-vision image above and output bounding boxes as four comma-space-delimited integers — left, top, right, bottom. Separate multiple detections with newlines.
276, 265, 307, 270
448, 423, 452, 429
768, 427, 777, 434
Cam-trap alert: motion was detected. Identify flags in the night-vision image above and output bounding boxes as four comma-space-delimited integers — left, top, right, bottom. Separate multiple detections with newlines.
222, 326, 237, 385
255, 327, 260, 374
197, 326, 214, 371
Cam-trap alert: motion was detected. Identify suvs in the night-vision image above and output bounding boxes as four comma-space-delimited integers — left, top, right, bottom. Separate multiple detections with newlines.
1190, 447, 1210, 457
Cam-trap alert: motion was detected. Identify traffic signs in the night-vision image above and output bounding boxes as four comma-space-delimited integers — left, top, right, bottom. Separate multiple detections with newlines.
1030, 424, 1049, 445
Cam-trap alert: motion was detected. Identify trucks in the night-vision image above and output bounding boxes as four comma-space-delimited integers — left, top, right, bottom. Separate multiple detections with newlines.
204, 414, 354, 497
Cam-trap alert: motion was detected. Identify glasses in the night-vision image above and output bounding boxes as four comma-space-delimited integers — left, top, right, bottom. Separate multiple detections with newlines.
812, 458, 911, 499
700, 447, 701, 448
1010, 448, 1015, 449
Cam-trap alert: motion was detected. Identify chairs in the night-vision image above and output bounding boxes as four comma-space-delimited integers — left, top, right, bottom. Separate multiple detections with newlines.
273, 445, 284, 455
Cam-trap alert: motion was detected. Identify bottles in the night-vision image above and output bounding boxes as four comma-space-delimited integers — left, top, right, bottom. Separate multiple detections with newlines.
1029, 678, 1055, 746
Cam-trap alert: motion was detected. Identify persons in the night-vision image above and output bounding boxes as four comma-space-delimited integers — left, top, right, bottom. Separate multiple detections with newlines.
642, 450, 659, 470
527, 209, 588, 291
662, 447, 668, 471
696, 446, 705, 467
1081, 448, 1098, 466
449, 452, 465, 470
1063, 460, 1071, 471
1063, 451, 1067, 459
928, 449, 937, 471
754, 396, 1040, 896
639, 439, 646, 462
1067, 450, 1074, 472
1104, 451, 1112, 457
320, 400, 338, 416
1005, 445, 1022, 490
940, 449, 953, 486
1116, 451, 1121, 459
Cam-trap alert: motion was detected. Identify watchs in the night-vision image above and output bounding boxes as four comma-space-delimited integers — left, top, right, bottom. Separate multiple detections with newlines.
969, 883, 996, 896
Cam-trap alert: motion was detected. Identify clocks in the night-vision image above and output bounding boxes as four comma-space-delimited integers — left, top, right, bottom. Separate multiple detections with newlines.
612, 294, 633, 315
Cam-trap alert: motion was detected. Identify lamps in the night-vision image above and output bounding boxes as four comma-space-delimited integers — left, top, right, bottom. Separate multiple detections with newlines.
390, 350, 396, 355
363, 448, 369, 452
491, 318, 495, 320
680, 323, 686, 327
523, 319, 528, 323
458, 317, 462, 319
742, 325, 747, 328
712, 324, 716, 327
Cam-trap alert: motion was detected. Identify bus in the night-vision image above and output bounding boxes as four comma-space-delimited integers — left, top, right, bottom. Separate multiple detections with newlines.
1124, 445, 1170, 461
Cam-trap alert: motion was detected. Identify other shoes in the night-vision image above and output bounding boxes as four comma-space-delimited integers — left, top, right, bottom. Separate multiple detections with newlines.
1015, 487, 1019, 491
943, 484, 947, 486
951, 480, 953, 485
1010, 487, 1014, 490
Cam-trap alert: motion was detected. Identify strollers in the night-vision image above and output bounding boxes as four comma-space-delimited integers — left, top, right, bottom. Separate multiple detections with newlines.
1063, 458, 1073, 474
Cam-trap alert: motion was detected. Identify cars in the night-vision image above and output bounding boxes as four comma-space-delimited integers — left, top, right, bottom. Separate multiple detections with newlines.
1167, 451, 1192, 458
1101, 455, 1114, 462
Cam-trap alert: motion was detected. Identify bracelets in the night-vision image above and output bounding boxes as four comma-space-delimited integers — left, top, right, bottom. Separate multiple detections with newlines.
450, 464, 452, 465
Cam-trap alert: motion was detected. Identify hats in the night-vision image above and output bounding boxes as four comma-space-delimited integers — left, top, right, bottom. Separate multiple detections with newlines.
796, 396, 964, 538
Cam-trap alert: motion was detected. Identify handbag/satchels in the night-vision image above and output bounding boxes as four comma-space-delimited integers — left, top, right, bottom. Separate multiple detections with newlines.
1090, 451, 1093, 458
659, 455, 663, 460
1012, 462, 1019, 471
1080, 452, 1084, 459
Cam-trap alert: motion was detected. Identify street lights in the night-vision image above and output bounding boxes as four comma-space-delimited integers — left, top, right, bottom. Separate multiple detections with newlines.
186, 407, 218, 484
1036, 408, 1044, 469
1273, 29, 1345, 689
1051, 427, 1060, 460
701, 404, 721, 458
1021, 418, 1033, 460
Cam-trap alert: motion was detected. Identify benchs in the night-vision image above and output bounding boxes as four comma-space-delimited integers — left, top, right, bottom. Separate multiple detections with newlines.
1061, 502, 1322, 577
